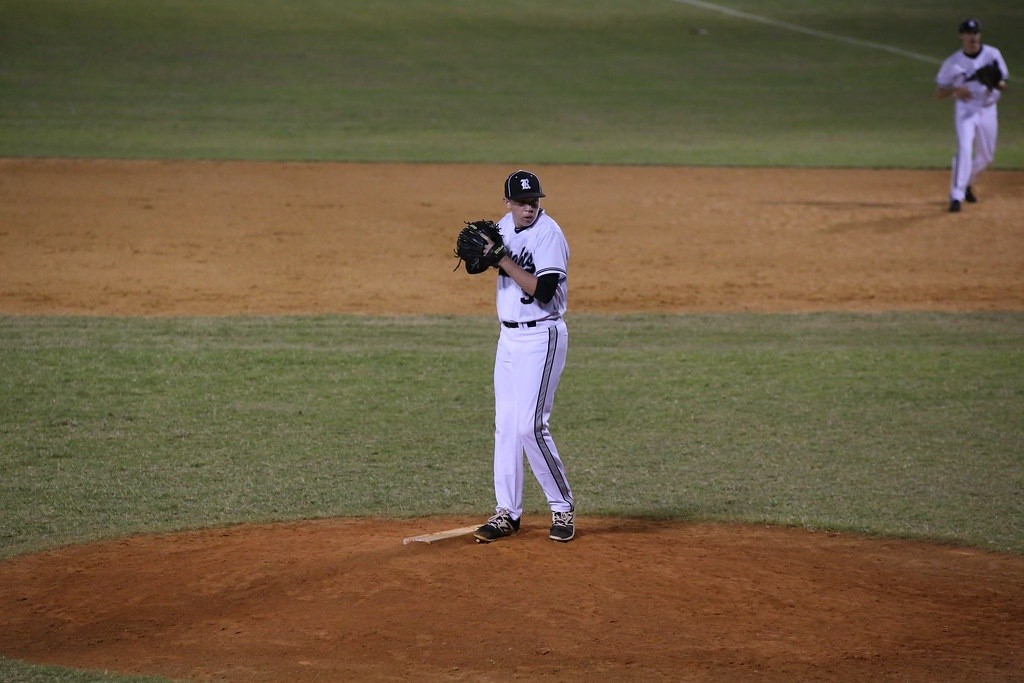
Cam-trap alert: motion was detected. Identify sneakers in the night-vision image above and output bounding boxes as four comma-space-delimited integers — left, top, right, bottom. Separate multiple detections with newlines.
475, 514, 519, 542
550, 510, 575, 541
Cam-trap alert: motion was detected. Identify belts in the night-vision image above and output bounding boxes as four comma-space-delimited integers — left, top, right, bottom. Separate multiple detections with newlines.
503, 318, 561, 328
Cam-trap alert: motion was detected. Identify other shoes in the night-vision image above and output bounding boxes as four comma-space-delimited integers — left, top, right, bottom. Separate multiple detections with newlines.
951, 200, 960, 212
965, 185, 975, 201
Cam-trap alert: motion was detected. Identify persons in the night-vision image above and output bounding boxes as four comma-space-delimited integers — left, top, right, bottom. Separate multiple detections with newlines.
462, 170, 577, 543
935, 16, 1009, 213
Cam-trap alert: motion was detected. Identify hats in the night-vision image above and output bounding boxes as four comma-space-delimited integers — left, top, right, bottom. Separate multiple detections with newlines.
960, 17, 982, 32
505, 169, 546, 199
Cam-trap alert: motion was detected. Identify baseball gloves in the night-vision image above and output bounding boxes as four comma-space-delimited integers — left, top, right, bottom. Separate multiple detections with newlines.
454, 219, 507, 274
